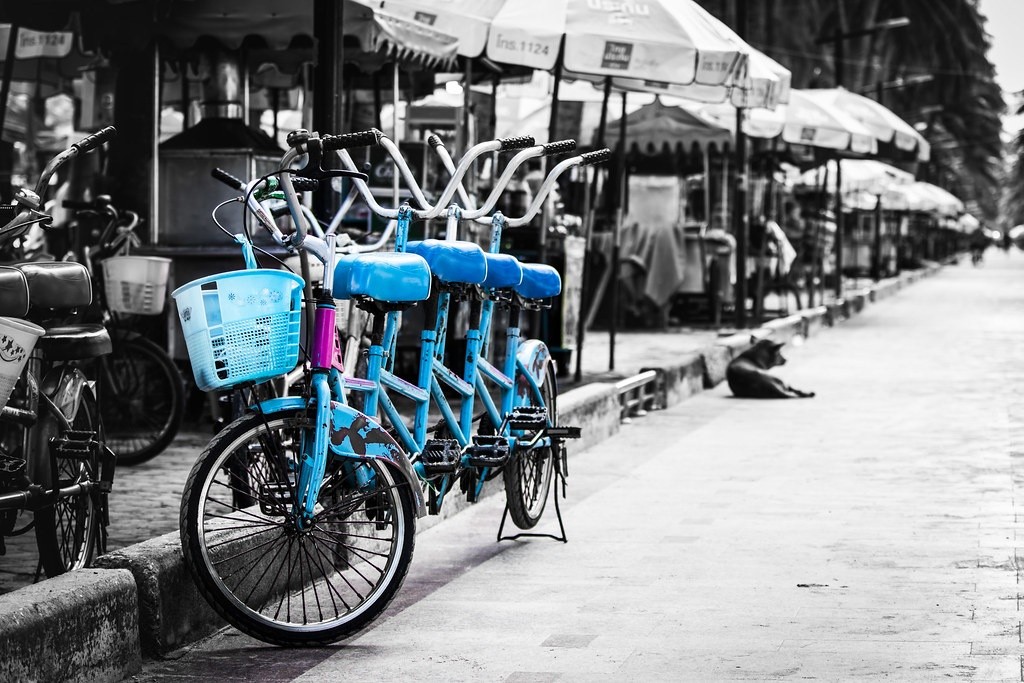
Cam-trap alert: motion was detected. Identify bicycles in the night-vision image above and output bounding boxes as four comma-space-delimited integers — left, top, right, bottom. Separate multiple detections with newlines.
0, 122, 320, 583
177, 123, 613, 648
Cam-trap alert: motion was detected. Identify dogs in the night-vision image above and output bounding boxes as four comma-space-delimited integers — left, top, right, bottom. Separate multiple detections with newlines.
727, 335, 815, 399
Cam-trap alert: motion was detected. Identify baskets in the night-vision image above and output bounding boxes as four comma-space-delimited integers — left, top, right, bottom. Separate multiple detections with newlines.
100, 257, 171, 315
170, 266, 305, 394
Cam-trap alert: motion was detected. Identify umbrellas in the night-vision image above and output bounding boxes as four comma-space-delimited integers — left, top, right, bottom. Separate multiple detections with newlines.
352, 0, 748, 401
542, 0, 965, 328
